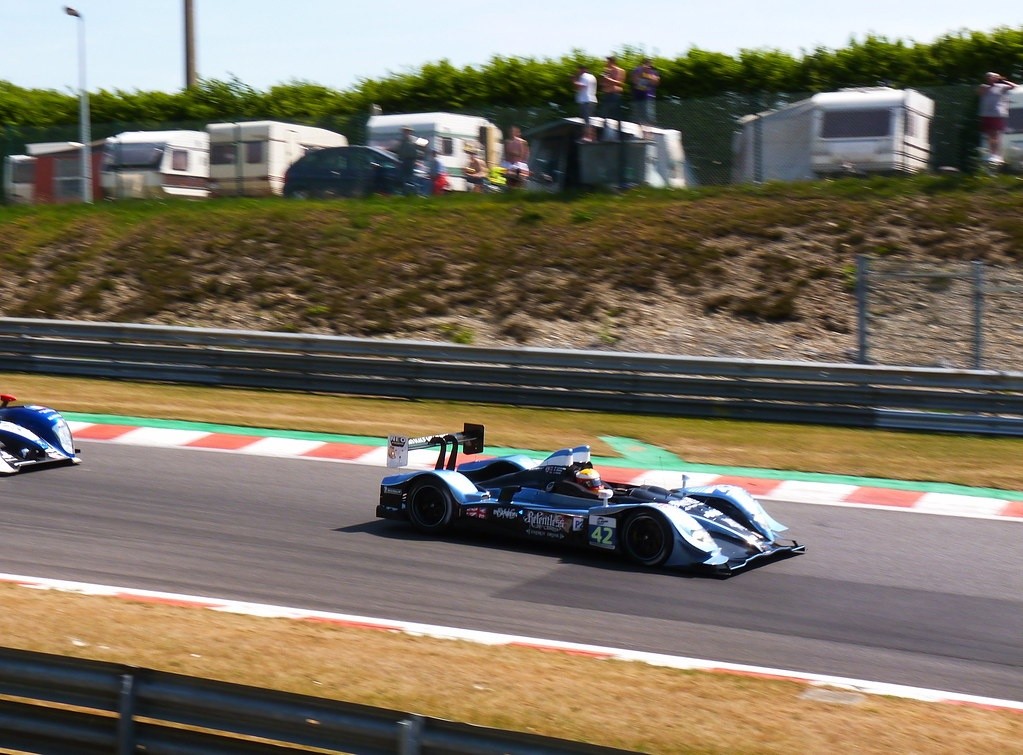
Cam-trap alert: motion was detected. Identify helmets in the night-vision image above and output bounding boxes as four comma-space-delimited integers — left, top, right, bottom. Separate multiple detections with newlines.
576, 468, 604, 495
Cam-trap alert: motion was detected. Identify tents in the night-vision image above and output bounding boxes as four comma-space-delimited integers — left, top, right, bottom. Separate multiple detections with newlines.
515, 116, 686, 192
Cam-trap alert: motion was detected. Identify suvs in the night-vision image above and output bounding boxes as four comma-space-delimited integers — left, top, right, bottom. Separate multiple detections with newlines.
283, 146, 435, 199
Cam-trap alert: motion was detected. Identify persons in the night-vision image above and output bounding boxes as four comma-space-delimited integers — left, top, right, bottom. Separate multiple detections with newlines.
501, 127, 529, 191
398, 125, 490, 193
573, 57, 660, 143
974, 71, 1017, 169
575, 469, 604, 497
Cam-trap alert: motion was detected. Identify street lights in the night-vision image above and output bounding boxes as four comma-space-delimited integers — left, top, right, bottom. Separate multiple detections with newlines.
63, 6, 92, 202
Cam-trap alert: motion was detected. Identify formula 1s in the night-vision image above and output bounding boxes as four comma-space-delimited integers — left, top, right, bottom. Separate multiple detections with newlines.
0, 393, 83, 475
374, 421, 806, 582
